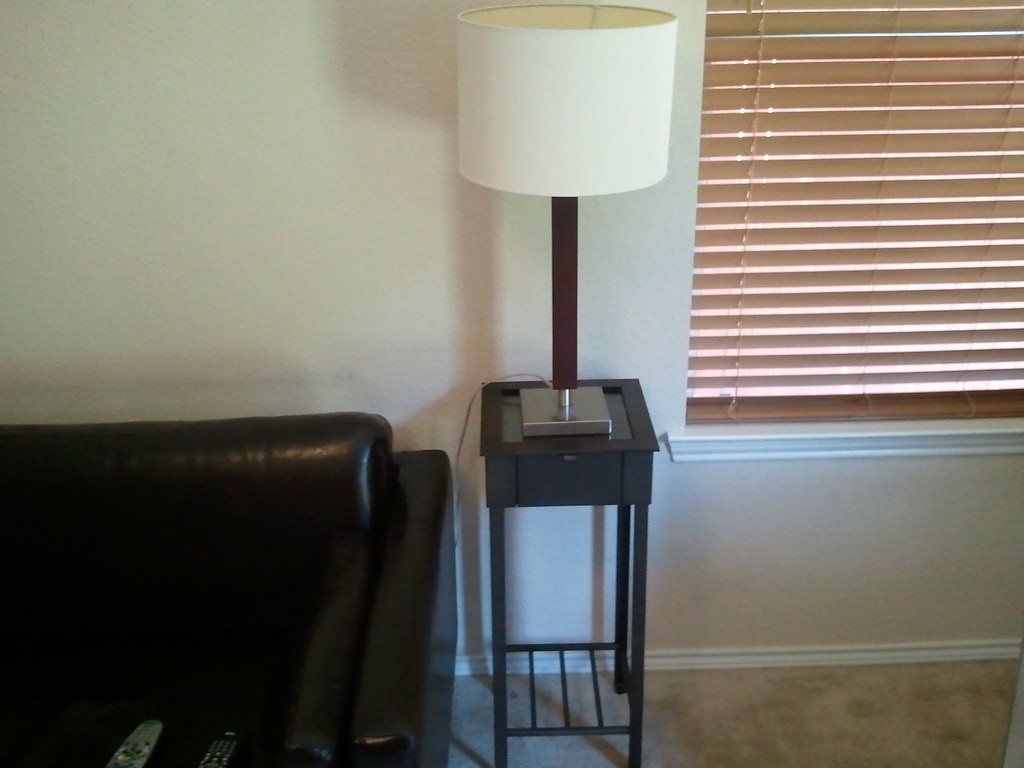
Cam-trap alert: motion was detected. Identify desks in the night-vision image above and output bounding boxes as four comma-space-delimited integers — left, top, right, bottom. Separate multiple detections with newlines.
475, 377, 660, 768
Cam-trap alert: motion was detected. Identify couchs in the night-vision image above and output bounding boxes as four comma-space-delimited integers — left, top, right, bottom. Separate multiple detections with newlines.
0, 416, 460, 768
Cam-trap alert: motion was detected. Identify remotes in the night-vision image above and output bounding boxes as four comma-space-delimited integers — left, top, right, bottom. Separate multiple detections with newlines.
105, 719, 163, 768
198, 730, 240, 768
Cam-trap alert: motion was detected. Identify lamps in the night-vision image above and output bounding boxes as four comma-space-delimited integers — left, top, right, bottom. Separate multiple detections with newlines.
455, 1, 680, 435
